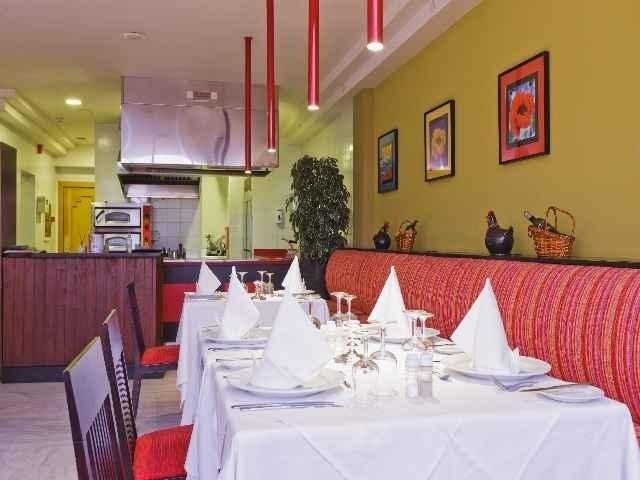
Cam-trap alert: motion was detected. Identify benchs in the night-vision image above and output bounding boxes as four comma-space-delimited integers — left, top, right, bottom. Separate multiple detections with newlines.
324, 247, 640, 445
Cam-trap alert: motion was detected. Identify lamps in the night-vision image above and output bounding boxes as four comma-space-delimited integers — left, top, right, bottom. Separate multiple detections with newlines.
266, 0, 276, 153
365, 0, 385, 52
307, 0, 321, 111
243, 36, 252, 172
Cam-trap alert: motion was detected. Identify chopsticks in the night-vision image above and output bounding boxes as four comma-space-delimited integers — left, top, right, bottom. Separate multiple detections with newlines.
442, 353, 552, 384
522, 383, 590, 392
232, 402, 346, 411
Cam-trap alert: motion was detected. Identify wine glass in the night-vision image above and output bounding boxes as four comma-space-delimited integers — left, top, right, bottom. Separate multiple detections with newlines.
353, 331, 381, 403
370, 327, 400, 398
400, 309, 424, 375
265, 273, 275, 296
257, 270, 268, 294
414, 313, 436, 373
238, 271, 249, 294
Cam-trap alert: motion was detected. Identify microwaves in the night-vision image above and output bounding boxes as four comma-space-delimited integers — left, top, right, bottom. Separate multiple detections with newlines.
92, 202, 144, 234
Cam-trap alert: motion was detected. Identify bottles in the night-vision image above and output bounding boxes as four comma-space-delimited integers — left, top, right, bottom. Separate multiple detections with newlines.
523, 210, 558, 236
404, 219, 419, 233
161, 241, 187, 262
102, 240, 111, 253
125, 235, 134, 254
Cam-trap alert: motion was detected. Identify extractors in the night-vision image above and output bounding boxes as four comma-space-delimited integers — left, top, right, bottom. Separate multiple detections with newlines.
113, 74, 279, 199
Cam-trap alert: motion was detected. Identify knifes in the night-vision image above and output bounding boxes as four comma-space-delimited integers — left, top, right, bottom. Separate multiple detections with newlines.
539, 379, 605, 405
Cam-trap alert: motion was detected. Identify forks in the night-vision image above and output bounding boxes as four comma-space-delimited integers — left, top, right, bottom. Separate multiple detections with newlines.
489, 373, 544, 393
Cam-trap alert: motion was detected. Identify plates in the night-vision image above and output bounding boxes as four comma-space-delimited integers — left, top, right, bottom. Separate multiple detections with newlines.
184, 279, 465, 376
226, 359, 349, 399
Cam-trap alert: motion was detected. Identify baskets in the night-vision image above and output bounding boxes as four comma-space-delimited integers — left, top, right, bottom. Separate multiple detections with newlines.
395, 220, 416, 251
528, 207, 576, 260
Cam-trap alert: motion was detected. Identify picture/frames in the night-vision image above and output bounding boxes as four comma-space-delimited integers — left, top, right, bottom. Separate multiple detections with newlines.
424, 99, 455, 182
378, 129, 398, 193
497, 51, 550, 164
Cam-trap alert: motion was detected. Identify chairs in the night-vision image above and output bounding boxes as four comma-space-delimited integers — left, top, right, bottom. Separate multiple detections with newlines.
63, 336, 128, 480
125, 279, 180, 420
100, 309, 194, 480
